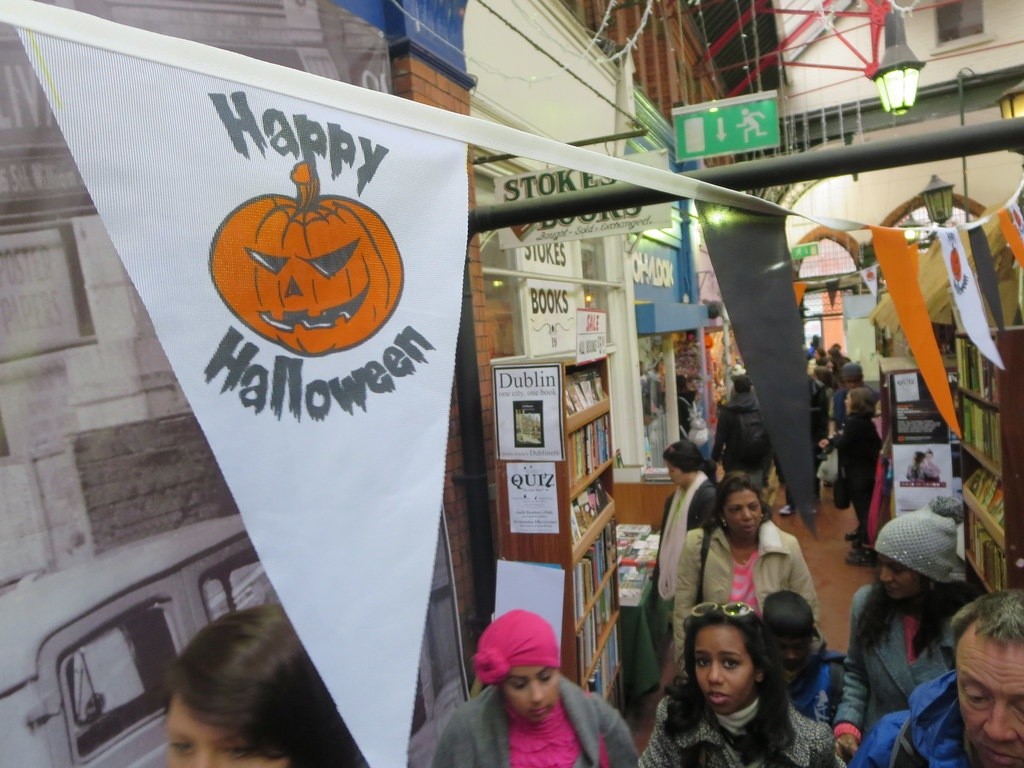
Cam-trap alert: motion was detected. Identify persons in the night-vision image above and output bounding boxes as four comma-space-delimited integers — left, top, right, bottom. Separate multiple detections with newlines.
674, 473, 820, 682
833, 497, 981, 759
761, 590, 847, 736
431, 610, 641, 768
636, 602, 848, 768
164, 601, 358, 768
849, 588, 1024, 768
653, 441, 718, 628
677, 336, 883, 566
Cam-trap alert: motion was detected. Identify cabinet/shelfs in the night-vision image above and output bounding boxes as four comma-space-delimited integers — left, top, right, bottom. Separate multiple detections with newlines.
952, 325, 1024, 597
494, 351, 628, 714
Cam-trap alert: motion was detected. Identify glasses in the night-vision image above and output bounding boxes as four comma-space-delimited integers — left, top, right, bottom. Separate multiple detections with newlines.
689, 602, 755, 619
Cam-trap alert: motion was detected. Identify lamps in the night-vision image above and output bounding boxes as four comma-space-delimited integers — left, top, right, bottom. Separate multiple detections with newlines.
871, 11, 1024, 228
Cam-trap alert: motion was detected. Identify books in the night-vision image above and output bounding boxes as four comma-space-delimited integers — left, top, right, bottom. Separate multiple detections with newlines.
953, 336, 1009, 594
561, 365, 627, 715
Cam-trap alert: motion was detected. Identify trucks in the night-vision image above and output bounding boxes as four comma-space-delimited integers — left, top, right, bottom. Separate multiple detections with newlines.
18, 527, 466, 768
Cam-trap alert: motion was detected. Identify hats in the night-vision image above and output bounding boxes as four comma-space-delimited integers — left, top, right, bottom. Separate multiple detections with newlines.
872, 493, 965, 582
734, 378, 751, 393
841, 363, 862, 377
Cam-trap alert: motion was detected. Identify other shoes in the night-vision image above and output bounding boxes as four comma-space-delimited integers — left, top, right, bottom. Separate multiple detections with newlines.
845, 545, 877, 567
778, 503, 792, 514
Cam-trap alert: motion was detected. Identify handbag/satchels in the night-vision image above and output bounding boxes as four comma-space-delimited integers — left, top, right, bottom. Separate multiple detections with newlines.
816, 448, 838, 484
832, 477, 851, 509
